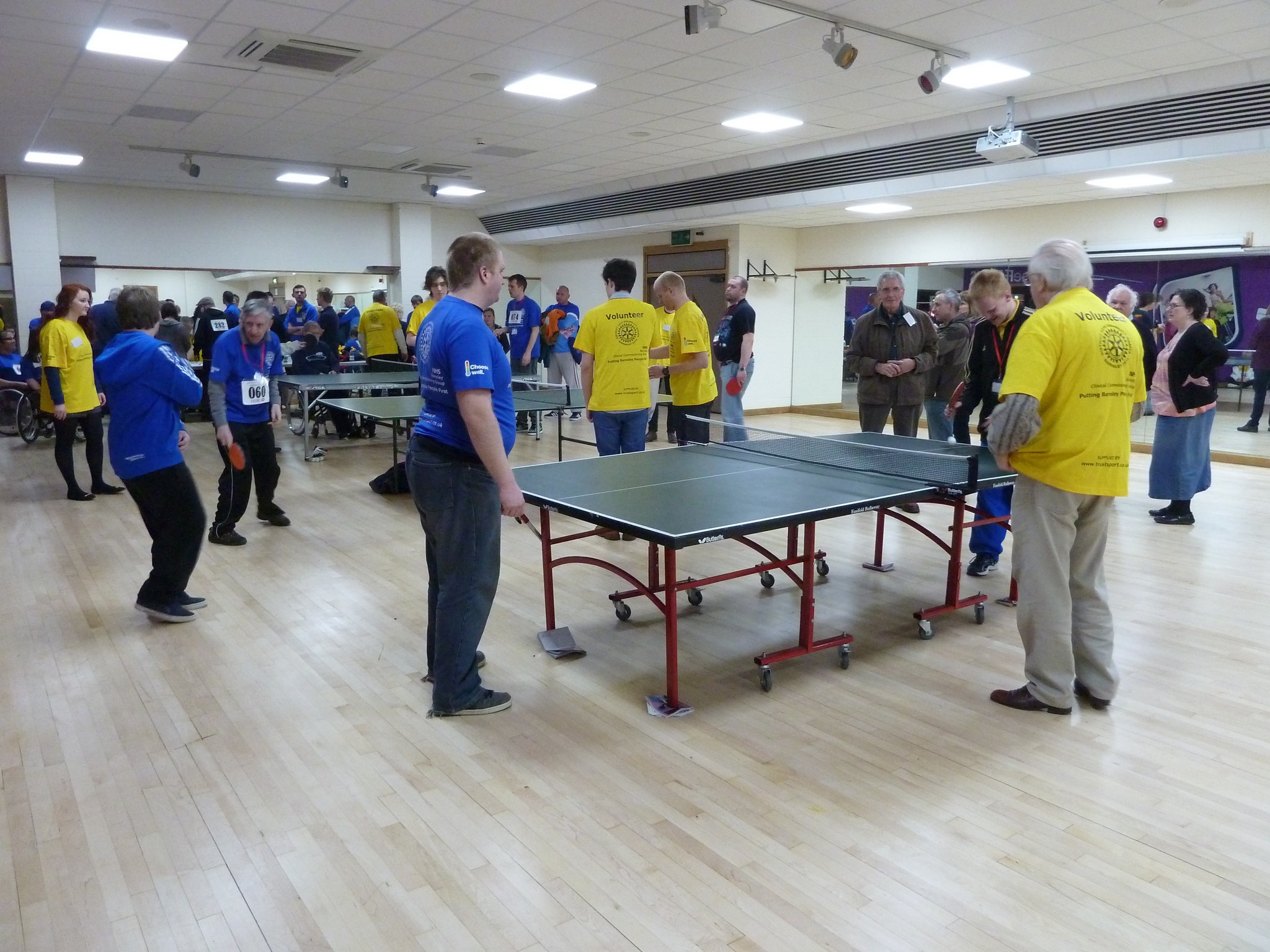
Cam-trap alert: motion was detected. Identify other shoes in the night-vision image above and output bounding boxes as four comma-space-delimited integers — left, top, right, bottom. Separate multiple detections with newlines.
622, 532, 636, 541
645, 433, 657, 442
91, 484, 125, 495
595, 525, 620, 540
135, 596, 196, 622
178, 592, 206, 610
1148, 504, 1176, 516
895, 503, 919, 513
1237, 420, 1258, 432
668, 433, 678, 443
67, 490, 96, 501
1154, 512, 1195, 525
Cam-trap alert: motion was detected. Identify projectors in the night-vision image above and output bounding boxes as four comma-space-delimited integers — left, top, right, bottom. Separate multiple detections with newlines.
975, 130, 1040, 163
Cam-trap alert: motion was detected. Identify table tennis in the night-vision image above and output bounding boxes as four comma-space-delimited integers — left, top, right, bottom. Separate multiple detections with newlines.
947, 436, 956, 445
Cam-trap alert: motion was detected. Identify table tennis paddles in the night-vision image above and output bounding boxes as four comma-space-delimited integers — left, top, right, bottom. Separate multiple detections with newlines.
945, 381, 964, 419
726, 377, 743, 396
229, 444, 245, 470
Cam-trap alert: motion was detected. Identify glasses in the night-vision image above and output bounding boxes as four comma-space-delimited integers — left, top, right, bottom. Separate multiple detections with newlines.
881, 288, 902, 293
1022, 273, 1036, 287
1167, 303, 1185, 309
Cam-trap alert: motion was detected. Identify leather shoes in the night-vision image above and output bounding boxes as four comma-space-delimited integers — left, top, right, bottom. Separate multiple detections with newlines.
990, 681, 1071, 715
1075, 676, 1109, 710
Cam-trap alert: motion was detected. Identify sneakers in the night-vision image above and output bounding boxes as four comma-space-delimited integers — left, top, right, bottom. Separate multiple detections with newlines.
967, 552, 999, 576
528, 422, 543, 435
544, 411, 563, 418
569, 412, 582, 420
428, 651, 485, 681
208, 527, 248, 546
434, 689, 511, 715
257, 509, 290, 526
516, 421, 529, 433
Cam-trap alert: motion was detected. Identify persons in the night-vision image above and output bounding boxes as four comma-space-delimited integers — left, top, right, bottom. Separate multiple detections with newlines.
39, 284, 125, 501
943, 269, 1036, 577
1236, 305, 1270, 432
199, 298, 291, 546
94, 285, 208, 623
404, 227, 526, 719
986, 237, 1146, 717
1142, 288, 1229, 526
0, 265, 1226, 514
648, 271, 719, 446
713, 275, 758, 445
573, 258, 663, 542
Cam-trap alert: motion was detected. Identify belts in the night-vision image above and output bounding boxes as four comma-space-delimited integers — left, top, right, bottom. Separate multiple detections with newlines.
721, 354, 754, 366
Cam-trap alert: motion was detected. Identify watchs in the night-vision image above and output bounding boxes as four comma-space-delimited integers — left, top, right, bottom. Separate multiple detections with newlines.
738, 367, 746, 372
663, 366, 671, 377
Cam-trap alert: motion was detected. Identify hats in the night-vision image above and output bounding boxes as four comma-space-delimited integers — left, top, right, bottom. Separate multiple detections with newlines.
196, 297, 214, 306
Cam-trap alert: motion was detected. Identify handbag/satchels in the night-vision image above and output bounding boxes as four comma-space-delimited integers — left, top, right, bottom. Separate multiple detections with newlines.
569, 337, 581, 365
